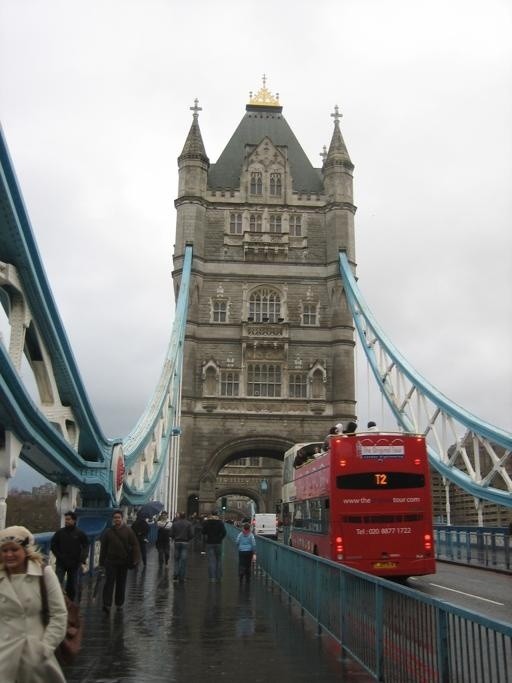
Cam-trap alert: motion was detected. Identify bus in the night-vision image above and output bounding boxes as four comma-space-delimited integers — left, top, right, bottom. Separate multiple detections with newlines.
280, 424, 437, 579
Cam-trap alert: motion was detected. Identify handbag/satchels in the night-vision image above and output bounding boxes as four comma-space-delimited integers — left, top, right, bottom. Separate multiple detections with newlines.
40, 565, 83, 661
127, 550, 139, 569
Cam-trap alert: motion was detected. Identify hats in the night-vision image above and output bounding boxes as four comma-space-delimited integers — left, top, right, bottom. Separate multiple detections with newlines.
0, 526, 33, 548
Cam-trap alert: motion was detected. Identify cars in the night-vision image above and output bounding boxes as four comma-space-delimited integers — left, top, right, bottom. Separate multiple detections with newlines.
226, 515, 251, 530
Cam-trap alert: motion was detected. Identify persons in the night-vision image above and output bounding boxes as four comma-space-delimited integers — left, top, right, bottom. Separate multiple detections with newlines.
131, 512, 150, 565
0, 525, 69, 683
171, 510, 227, 583
293, 421, 380, 469
49, 511, 90, 601
153, 511, 173, 570
235, 523, 257, 585
98, 510, 142, 614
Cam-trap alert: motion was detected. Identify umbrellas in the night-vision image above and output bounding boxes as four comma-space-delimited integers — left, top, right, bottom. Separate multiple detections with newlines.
136, 501, 164, 519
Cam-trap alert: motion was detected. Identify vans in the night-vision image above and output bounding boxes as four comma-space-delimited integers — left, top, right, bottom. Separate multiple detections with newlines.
250, 513, 279, 540
